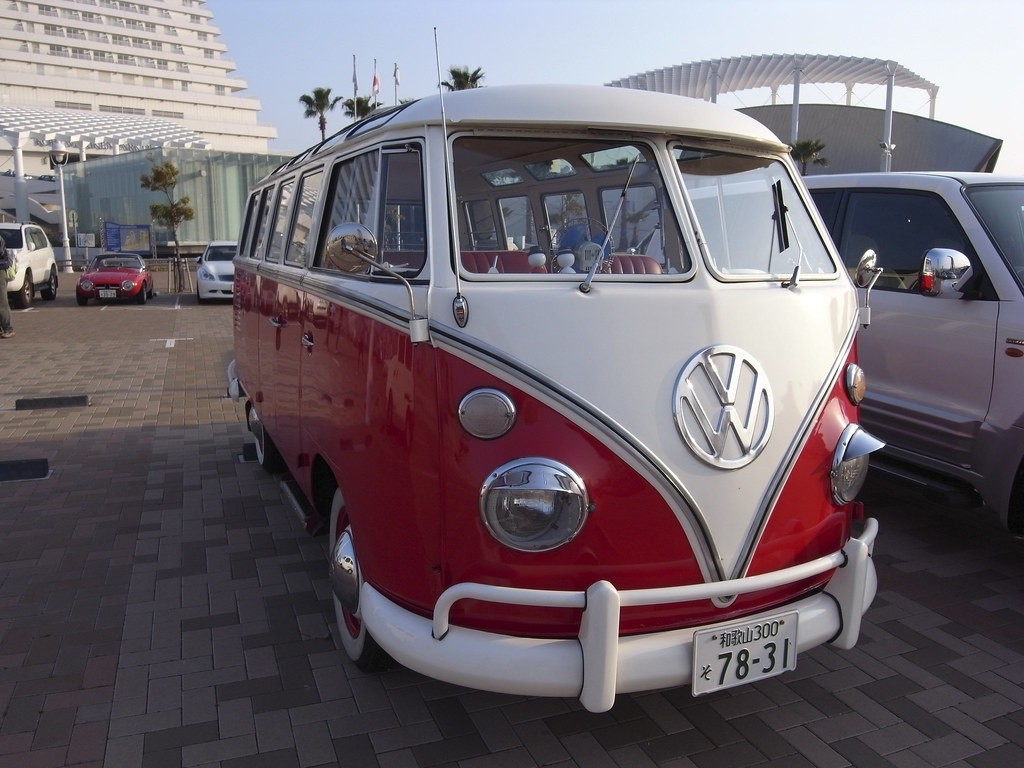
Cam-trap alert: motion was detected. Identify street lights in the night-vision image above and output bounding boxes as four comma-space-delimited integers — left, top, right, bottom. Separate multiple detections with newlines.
48, 142, 75, 274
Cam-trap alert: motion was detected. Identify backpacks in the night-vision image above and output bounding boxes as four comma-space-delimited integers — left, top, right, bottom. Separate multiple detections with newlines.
7, 256, 20, 282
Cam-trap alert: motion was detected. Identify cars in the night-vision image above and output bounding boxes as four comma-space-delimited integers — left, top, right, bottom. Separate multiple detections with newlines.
76, 252, 154, 305
194, 239, 237, 304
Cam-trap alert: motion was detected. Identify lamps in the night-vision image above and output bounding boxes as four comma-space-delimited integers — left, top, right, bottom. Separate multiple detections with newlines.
879, 141, 888, 150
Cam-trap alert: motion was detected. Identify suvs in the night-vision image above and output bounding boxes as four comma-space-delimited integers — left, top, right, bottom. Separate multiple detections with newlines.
0, 220, 59, 310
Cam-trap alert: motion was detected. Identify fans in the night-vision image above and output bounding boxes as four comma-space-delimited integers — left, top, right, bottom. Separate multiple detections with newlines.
549, 218, 615, 275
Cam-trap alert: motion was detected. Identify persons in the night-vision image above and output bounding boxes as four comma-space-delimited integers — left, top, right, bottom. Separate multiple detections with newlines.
341, 235, 373, 264
0, 234, 16, 338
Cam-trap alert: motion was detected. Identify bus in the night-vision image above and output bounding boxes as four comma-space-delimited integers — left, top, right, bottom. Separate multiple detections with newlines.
226, 82, 896, 715
639, 167, 1024, 542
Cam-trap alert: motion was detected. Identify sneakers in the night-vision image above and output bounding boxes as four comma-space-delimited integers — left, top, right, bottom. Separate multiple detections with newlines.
0, 327, 3, 334
1, 329, 15, 337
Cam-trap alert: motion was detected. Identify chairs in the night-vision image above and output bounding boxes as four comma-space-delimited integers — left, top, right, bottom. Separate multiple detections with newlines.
846, 233, 907, 289
382, 250, 664, 276
